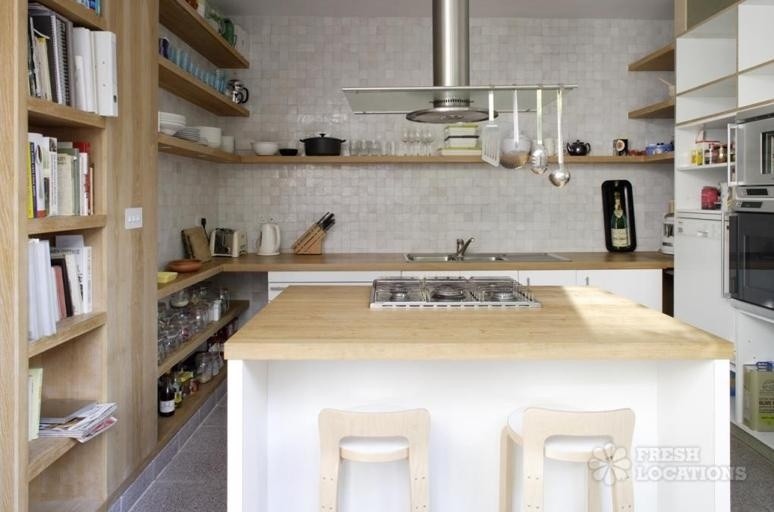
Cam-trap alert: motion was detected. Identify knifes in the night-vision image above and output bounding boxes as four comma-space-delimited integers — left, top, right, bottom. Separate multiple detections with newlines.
318, 210, 336, 234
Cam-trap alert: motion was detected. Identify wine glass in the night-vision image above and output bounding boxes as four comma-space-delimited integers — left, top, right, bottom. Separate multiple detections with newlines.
400, 128, 435, 157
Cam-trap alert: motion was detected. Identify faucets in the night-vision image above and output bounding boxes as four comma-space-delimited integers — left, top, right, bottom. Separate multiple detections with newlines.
455, 237, 475, 261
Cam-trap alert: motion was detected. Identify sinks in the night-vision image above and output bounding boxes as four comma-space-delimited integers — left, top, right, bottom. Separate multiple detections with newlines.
457, 254, 511, 261
403, 253, 456, 262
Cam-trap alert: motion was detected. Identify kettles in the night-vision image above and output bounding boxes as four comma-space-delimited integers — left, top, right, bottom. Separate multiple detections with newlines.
254, 222, 280, 256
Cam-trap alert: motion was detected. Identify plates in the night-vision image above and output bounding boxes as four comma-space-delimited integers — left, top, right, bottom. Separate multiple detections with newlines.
159, 112, 221, 150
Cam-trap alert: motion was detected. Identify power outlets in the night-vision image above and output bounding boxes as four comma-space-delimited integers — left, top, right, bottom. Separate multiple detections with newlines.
195, 213, 208, 229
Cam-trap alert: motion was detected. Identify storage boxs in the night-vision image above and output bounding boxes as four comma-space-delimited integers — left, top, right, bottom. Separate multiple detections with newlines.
742, 361, 774, 435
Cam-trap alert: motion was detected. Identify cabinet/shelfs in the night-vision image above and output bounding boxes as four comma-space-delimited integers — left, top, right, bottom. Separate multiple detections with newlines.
156, 0, 251, 439
624, 41, 676, 122
0, 0, 113, 512
222, 146, 673, 273
518, 270, 578, 288
578, 269, 662, 315
665, 0, 774, 460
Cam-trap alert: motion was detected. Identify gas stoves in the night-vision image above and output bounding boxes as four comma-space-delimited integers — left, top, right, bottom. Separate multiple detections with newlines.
366, 276, 542, 311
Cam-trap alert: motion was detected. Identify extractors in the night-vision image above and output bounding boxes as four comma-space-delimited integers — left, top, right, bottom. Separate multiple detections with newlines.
333, 1, 572, 125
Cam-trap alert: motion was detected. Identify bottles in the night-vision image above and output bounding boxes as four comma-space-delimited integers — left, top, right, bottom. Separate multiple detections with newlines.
171, 369, 182, 408
610, 190, 630, 248
662, 201, 674, 256
195, 353, 212, 384
222, 17, 234, 47
157, 285, 230, 365
158, 373, 175, 416
701, 185, 717, 209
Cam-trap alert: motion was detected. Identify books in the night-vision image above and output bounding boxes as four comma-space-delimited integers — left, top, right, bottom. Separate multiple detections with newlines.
27, 1, 122, 448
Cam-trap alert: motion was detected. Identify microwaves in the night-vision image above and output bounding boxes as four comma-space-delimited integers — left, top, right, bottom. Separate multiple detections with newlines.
725, 121, 774, 187
726, 215, 773, 312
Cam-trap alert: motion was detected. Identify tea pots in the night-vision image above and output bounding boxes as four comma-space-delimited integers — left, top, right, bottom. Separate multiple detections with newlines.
567, 140, 591, 156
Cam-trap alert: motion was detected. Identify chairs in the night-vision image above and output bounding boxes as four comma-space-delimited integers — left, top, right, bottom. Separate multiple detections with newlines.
315, 404, 434, 512
500, 398, 643, 512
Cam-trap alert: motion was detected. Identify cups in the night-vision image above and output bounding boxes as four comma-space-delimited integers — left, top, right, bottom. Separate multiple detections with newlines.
347, 139, 398, 157
690, 146, 702, 165
617, 139, 628, 157
220, 135, 234, 154
158, 38, 224, 93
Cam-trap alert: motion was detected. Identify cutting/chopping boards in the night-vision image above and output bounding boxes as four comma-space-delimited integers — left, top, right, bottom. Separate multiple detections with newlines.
180, 226, 212, 262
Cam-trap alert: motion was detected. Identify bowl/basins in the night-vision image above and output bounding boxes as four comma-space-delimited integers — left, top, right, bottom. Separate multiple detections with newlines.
250, 141, 280, 156
169, 259, 201, 272
645, 145, 672, 156
278, 148, 298, 156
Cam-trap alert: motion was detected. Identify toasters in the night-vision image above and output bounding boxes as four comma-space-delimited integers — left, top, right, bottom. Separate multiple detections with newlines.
208, 228, 248, 258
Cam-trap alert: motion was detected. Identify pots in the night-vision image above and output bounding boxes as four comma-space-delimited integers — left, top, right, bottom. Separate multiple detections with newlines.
299, 134, 346, 154
